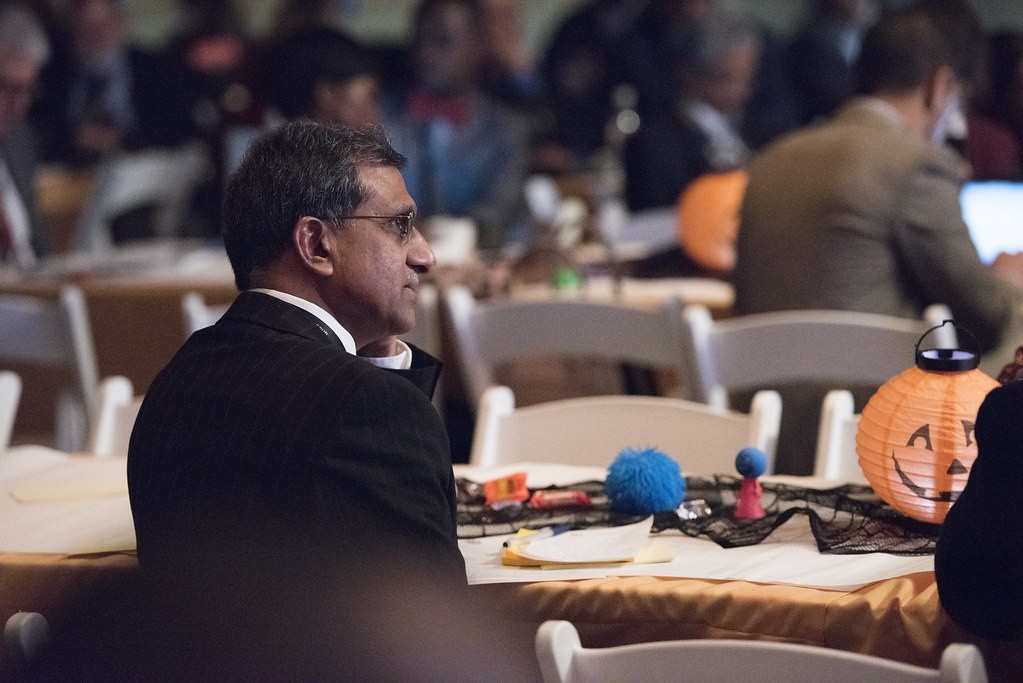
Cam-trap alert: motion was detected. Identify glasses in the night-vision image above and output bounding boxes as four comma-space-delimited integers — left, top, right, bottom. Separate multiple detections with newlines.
314, 208, 417, 243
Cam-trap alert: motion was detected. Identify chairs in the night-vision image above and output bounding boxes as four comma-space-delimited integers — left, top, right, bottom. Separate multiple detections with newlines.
441, 284, 683, 383
681, 304, 960, 483
1, 148, 241, 457
535, 620, 987, 683
468, 386, 783, 475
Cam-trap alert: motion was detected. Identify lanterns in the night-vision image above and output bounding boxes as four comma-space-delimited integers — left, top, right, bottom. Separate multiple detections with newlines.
673, 169, 752, 273
858, 320, 1004, 524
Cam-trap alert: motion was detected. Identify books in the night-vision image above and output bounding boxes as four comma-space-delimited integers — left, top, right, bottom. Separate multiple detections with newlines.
502, 528, 634, 567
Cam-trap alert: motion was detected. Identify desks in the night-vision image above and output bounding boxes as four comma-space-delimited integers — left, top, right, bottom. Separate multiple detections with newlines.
0, 463, 941, 667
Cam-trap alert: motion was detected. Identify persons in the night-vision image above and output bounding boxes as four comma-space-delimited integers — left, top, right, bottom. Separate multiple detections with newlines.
127, 120, 539, 683
935, 379, 1023, 642
0, 0, 1023, 476
